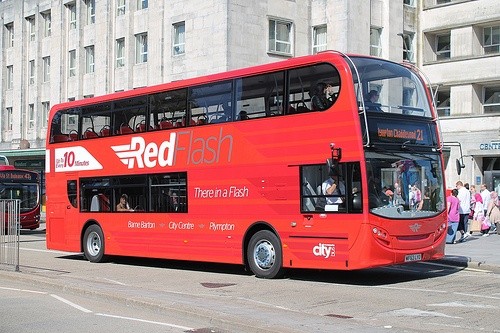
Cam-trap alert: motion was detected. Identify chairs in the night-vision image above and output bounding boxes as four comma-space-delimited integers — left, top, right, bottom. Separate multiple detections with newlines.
85, 127, 98, 139
100, 125, 110, 136
68, 130, 78, 140
120, 122, 134, 134
311, 95, 321, 110
297, 101, 307, 112
52, 135, 68, 142
136, 123, 142, 133
210, 114, 226, 123
197, 115, 207, 124
160, 117, 172, 129
140, 119, 153, 132
176, 116, 182, 127
183, 115, 195, 127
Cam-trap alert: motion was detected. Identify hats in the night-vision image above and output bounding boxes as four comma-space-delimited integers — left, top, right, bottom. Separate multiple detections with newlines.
386, 190, 394, 196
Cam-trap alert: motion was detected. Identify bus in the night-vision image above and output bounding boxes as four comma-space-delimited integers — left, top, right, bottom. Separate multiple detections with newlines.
0, 164, 44, 235
42, 49, 468, 280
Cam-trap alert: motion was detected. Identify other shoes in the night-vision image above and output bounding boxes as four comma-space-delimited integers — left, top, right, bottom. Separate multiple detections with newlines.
482, 234, 489, 236
459, 233, 468, 242
467, 232, 471, 237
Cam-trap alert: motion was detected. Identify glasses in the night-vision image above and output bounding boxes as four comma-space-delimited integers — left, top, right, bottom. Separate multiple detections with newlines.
456, 185, 458, 187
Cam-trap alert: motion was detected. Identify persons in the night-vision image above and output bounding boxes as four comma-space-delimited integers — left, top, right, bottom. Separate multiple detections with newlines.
169, 193, 181, 212
483, 191, 500, 235
320, 169, 358, 212
383, 181, 437, 211
312, 83, 335, 111
468, 194, 487, 236
463, 183, 476, 210
455, 181, 471, 242
365, 90, 384, 112
115, 194, 135, 211
445, 189, 460, 243
95, 188, 111, 211
478, 185, 491, 218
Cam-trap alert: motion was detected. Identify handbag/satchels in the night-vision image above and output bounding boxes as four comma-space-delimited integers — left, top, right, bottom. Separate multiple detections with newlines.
469, 220, 481, 231
482, 218, 489, 230
446, 222, 458, 244
